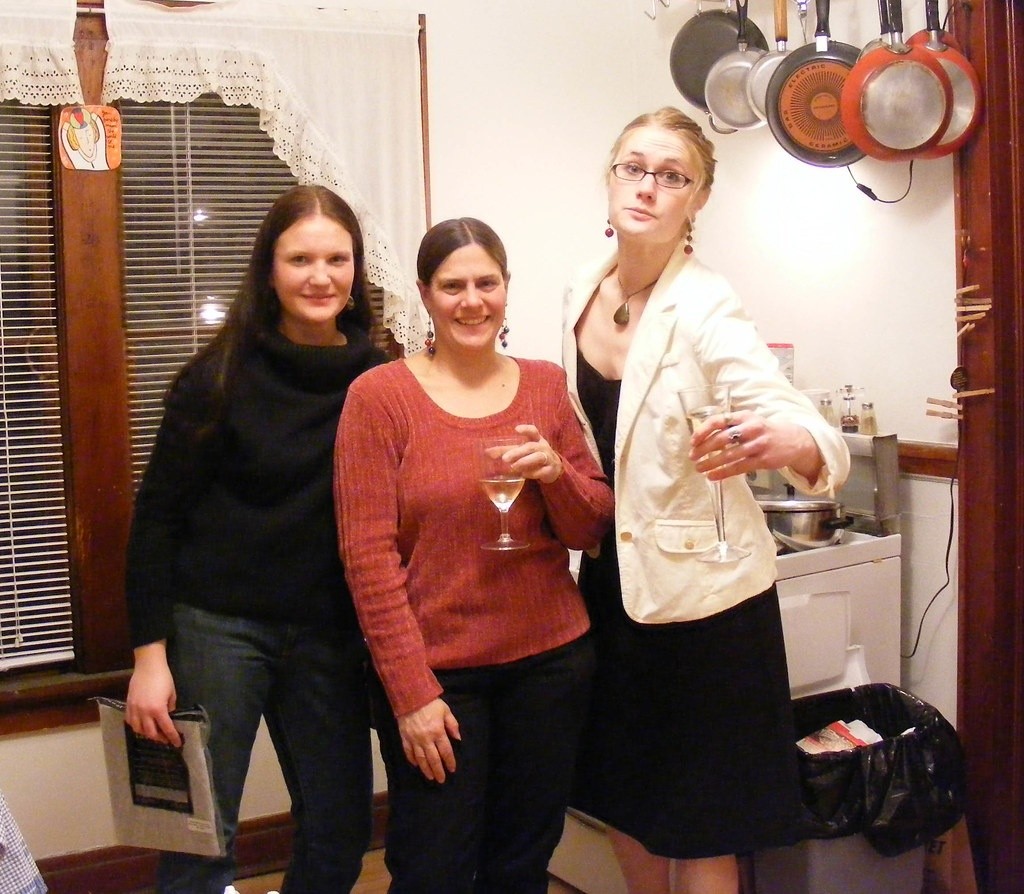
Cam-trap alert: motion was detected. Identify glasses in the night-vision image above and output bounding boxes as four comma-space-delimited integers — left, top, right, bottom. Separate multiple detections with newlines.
610, 163, 693, 189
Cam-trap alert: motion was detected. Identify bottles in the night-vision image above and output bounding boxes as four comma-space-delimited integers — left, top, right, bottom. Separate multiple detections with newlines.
860, 401, 878, 435
816, 400, 835, 427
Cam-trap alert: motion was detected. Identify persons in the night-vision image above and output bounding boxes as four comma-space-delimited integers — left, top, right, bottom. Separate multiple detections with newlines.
124, 186, 391, 893
553, 107, 851, 894
332, 217, 615, 894
614, 271, 658, 325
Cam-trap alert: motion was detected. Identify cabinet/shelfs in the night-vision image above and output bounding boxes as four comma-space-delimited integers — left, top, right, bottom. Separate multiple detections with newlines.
546, 530, 904, 894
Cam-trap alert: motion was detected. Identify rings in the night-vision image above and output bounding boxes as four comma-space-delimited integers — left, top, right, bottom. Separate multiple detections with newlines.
729, 427, 741, 444
723, 412, 733, 426
541, 452, 548, 465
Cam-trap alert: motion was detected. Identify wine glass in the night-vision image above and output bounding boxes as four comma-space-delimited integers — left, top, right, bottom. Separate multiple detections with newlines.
474, 433, 531, 551
678, 383, 752, 565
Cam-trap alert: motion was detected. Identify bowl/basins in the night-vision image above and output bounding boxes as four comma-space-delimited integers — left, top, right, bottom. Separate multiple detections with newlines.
751, 482, 855, 554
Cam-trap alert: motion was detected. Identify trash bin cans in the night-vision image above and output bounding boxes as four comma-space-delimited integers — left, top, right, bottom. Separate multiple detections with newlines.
752, 681, 944, 894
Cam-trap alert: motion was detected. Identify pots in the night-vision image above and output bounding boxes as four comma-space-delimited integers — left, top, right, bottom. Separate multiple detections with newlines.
668, 0, 980, 168
